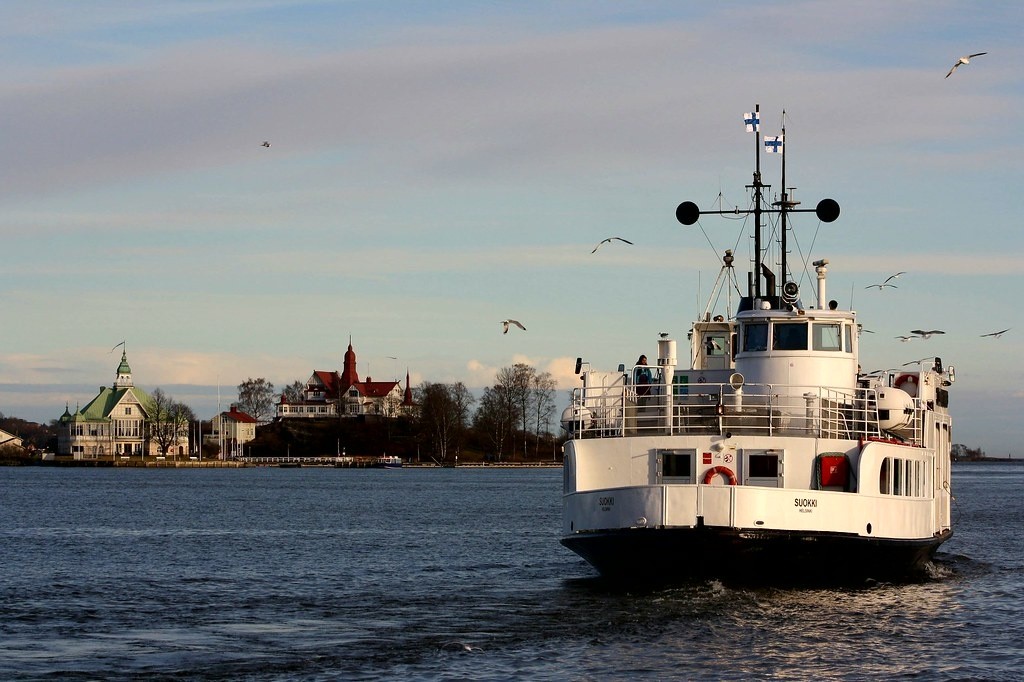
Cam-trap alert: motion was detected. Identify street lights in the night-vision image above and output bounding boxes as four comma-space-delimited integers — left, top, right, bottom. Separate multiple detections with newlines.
338, 437, 340, 457
287, 444, 291, 463
249, 446, 251, 456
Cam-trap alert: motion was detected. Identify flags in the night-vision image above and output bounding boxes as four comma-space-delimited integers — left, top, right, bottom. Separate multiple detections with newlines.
744, 112, 760, 133
764, 135, 783, 154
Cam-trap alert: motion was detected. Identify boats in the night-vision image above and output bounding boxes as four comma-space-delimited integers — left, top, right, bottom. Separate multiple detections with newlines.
279, 462, 300, 468
560, 104, 955, 589
373, 456, 403, 467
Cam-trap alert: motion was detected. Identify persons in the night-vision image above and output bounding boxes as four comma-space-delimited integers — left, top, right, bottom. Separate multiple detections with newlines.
634, 355, 652, 414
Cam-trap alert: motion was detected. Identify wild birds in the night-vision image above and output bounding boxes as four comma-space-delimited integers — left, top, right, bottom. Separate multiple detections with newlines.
902, 357, 934, 366
945, 52, 987, 78
862, 329, 875, 333
896, 329, 945, 341
979, 328, 1011, 340
870, 368, 900, 374
500, 319, 527, 334
863, 272, 905, 290
592, 237, 633, 253
435, 642, 486, 661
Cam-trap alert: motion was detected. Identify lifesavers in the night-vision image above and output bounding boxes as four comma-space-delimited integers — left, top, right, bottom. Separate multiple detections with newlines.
704, 465, 738, 487
894, 374, 919, 398
